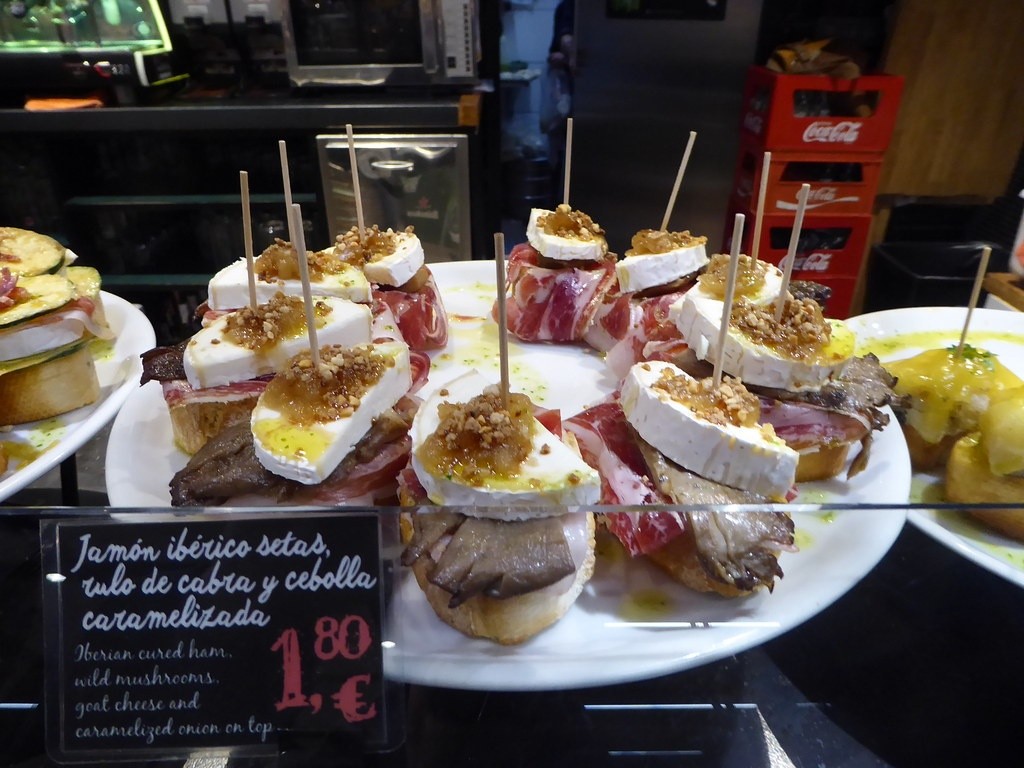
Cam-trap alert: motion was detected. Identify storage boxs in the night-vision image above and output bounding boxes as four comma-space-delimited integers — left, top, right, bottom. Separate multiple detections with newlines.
714, 64, 906, 322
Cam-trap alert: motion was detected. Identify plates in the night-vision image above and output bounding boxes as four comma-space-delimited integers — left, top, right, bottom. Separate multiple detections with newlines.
845, 307, 1024, 589
103, 260, 913, 691
0, 291, 158, 503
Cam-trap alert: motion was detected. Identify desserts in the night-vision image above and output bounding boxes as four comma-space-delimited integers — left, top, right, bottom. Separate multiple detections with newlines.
884, 345, 1024, 538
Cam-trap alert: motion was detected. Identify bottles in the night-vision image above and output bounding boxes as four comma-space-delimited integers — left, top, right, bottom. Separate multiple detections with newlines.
784, 162, 862, 183
752, 92, 872, 118
769, 228, 849, 253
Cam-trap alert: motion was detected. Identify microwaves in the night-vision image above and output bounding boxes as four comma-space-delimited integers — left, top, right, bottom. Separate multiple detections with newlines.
278, 0, 482, 89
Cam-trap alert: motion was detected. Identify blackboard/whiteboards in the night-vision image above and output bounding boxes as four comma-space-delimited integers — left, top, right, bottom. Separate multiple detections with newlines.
39, 505, 387, 766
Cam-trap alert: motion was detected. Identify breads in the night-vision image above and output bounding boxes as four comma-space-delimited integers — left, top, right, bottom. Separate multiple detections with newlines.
163, 394, 857, 648
0, 347, 104, 428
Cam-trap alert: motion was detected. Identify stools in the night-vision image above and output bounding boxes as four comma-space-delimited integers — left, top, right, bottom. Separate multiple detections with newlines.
859, 239, 1009, 315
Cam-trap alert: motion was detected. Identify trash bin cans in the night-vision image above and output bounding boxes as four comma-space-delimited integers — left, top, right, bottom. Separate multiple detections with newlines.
862, 238, 1011, 312
